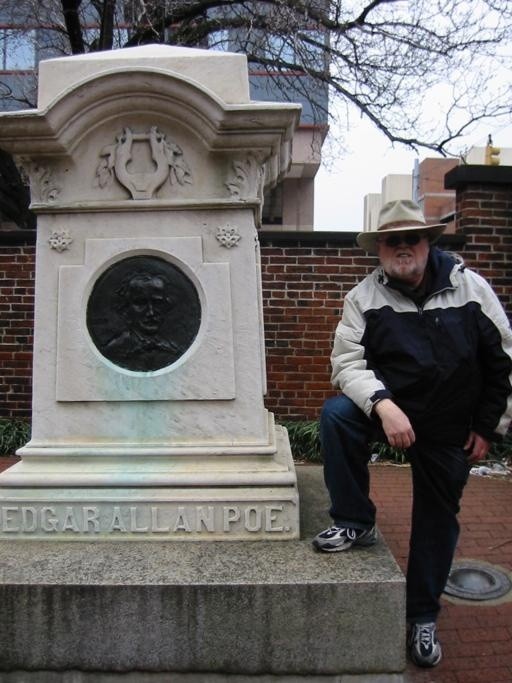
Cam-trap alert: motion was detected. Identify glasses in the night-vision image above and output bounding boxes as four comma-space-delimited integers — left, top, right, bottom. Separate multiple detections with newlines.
379, 233, 421, 246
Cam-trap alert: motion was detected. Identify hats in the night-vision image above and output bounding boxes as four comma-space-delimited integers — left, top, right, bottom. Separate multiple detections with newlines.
357, 200, 447, 252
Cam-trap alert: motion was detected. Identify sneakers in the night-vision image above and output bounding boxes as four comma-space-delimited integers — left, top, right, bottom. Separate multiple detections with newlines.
313, 524, 377, 551
409, 623, 442, 667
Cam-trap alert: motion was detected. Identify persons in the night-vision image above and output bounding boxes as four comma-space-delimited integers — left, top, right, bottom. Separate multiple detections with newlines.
101, 268, 186, 370
313, 199, 511, 667
